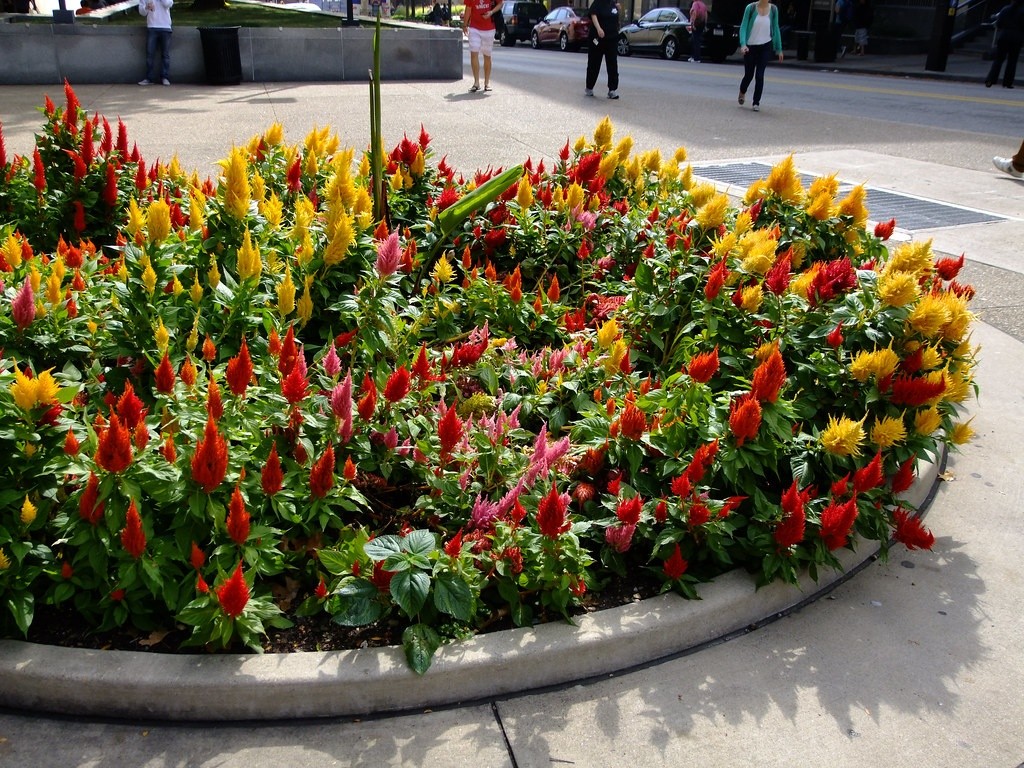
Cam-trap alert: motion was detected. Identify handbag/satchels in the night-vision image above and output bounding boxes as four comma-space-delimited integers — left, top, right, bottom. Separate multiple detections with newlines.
492, 0, 506, 33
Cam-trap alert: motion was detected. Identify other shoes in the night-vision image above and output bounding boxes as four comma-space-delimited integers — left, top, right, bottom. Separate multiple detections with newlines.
841, 45, 848, 58
161, 78, 171, 85
688, 57, 701, 63
753, 104, 759, 111
738, 91, 745, 105
138, 79, 155, 85
585, 88, 594, 96
608, 91, 619, 99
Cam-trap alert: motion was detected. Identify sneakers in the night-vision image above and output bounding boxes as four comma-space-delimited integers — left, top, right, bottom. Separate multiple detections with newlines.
993, 156, 1024, 178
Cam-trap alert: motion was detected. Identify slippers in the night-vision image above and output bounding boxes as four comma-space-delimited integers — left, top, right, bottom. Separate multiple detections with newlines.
468, 85, 481, 91
485, 85, 492, 91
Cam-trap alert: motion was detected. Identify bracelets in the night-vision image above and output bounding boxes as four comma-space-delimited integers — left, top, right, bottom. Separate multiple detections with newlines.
491, 11, 494, 15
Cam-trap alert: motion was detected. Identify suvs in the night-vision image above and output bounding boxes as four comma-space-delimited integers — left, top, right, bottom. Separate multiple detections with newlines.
494, 1, 548, 47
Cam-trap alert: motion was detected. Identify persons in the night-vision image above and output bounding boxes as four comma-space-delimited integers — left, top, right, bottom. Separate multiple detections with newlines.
351, 0, 503, 90
738, 0, 784, 111
687, 0, 707, 63
992, 139, 1024, 178
585, 0, 621, 99
985, 0, 1024, 88
138, 0, 173, 86
780, 0, 868, 58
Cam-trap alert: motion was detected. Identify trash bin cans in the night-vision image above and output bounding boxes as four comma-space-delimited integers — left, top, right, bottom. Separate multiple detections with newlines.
811, 22, 842, 63
196, 24, 244, 86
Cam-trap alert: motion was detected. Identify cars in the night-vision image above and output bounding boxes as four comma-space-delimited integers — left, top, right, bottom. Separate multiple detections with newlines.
618, 6, 740, 60
531, 5, 591, 51
